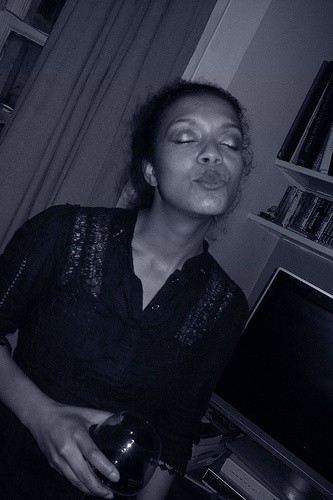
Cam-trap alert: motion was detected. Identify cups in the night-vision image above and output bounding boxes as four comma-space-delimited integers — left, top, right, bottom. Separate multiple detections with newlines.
78, 410, 162, 500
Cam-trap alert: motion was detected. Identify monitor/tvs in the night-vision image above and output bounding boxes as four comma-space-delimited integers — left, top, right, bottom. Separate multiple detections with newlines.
212, 267, 333, 500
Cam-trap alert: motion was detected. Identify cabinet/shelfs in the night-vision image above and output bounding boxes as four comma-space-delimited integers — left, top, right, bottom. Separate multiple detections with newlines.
246, 60, 333, 258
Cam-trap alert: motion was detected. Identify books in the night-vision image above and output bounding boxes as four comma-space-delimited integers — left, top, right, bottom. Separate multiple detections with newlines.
274, 61, 333, 249
186, 417, 222, 471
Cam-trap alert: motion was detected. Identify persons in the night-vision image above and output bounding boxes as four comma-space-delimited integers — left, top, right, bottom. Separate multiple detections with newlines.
0, 81, 249, 500
